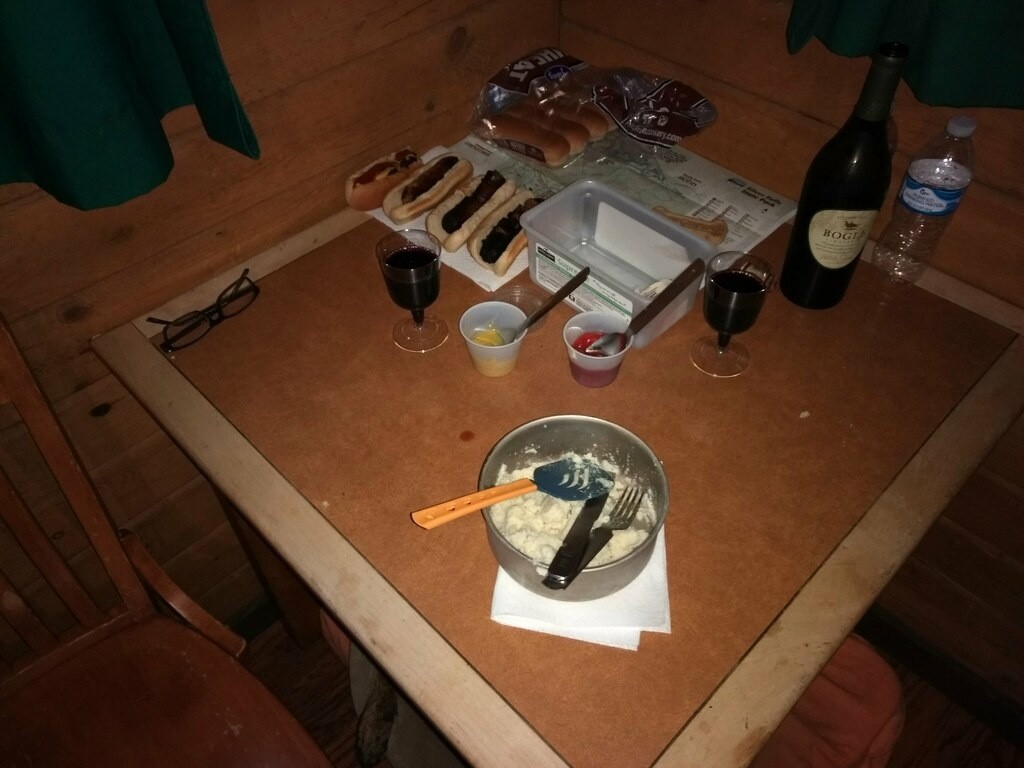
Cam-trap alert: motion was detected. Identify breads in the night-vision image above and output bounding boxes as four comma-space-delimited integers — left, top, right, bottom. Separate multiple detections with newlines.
478, 81, 626, 167
652, 206, 728, 244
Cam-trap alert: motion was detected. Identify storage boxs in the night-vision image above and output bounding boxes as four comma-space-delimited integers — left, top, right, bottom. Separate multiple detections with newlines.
518, 179, 714, 349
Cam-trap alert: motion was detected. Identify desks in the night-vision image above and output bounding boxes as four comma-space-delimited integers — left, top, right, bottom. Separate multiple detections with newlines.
90, 136, 1024, 768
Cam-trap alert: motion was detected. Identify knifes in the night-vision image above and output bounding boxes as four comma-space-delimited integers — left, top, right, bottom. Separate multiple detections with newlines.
548, 469, 615, 578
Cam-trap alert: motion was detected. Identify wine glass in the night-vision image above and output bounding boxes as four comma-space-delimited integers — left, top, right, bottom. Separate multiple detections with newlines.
690, 251, 774, 377
376, 228, 451, 352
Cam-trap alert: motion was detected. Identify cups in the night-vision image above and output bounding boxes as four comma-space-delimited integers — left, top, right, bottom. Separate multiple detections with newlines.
563, 312, 634, 388
459, 299, 530, 377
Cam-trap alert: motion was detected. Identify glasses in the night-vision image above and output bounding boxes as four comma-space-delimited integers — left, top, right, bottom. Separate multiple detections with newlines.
146, 268, 259, 354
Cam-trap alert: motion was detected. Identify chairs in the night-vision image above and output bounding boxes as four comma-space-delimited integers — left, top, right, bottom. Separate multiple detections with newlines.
0, 314, 332, 768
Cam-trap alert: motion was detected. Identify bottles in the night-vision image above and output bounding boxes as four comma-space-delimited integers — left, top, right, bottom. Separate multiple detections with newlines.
870, 112, 977, 286
780, 37, 911, 310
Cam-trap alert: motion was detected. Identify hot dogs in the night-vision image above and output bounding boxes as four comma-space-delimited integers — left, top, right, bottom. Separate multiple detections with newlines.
345, 146, 547, 277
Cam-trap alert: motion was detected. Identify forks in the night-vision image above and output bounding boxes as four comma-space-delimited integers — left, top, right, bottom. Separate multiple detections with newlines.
542, 486, 644, 590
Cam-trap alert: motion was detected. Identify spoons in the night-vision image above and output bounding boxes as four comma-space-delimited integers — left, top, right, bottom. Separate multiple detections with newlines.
497, 266, 590, 346
585, 256, 705, 356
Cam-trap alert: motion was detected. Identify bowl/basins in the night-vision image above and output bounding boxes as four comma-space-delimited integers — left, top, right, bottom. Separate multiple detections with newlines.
477, 414, 670, 601
